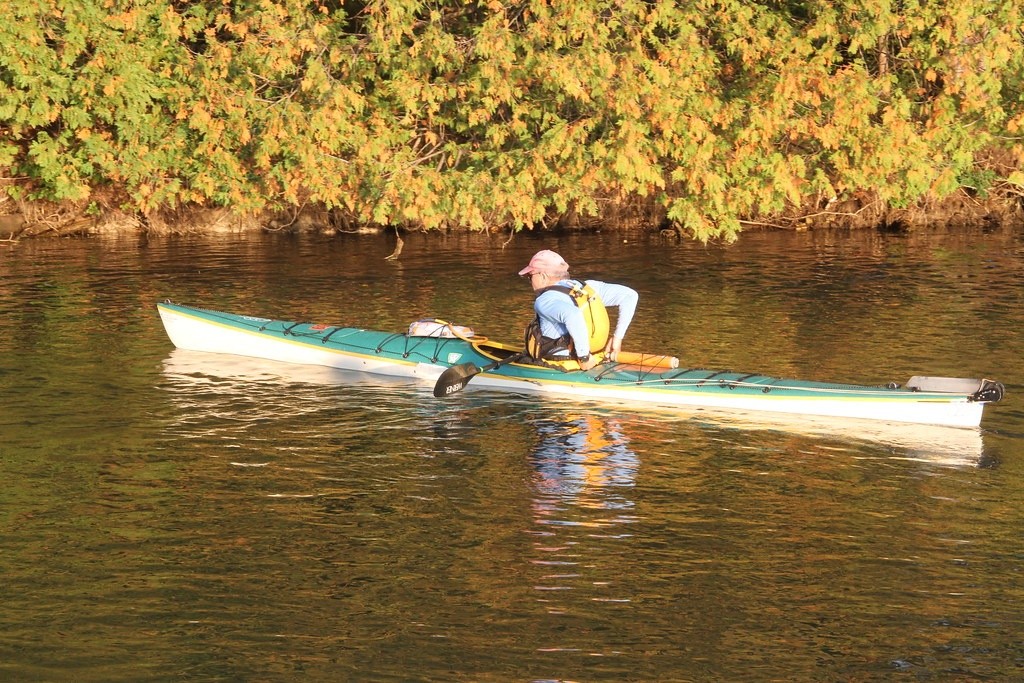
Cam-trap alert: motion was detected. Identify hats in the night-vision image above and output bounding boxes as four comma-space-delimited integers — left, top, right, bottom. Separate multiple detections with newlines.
518, 250, 569, 276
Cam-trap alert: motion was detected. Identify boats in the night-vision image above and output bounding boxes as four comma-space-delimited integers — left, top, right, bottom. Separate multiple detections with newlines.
156, 298, 1004, 430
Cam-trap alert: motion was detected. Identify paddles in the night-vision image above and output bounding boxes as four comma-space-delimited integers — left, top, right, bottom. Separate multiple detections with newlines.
433, 350, 529, 400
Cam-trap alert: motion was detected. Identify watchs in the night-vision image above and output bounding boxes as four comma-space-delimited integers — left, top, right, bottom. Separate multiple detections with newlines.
577, 353, 590, 362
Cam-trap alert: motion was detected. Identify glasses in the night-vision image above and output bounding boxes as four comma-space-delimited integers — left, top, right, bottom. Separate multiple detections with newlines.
528, 272, 541, 281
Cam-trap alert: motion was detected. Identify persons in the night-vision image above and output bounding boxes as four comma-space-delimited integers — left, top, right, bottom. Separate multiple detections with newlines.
527, 249, 640, 373
